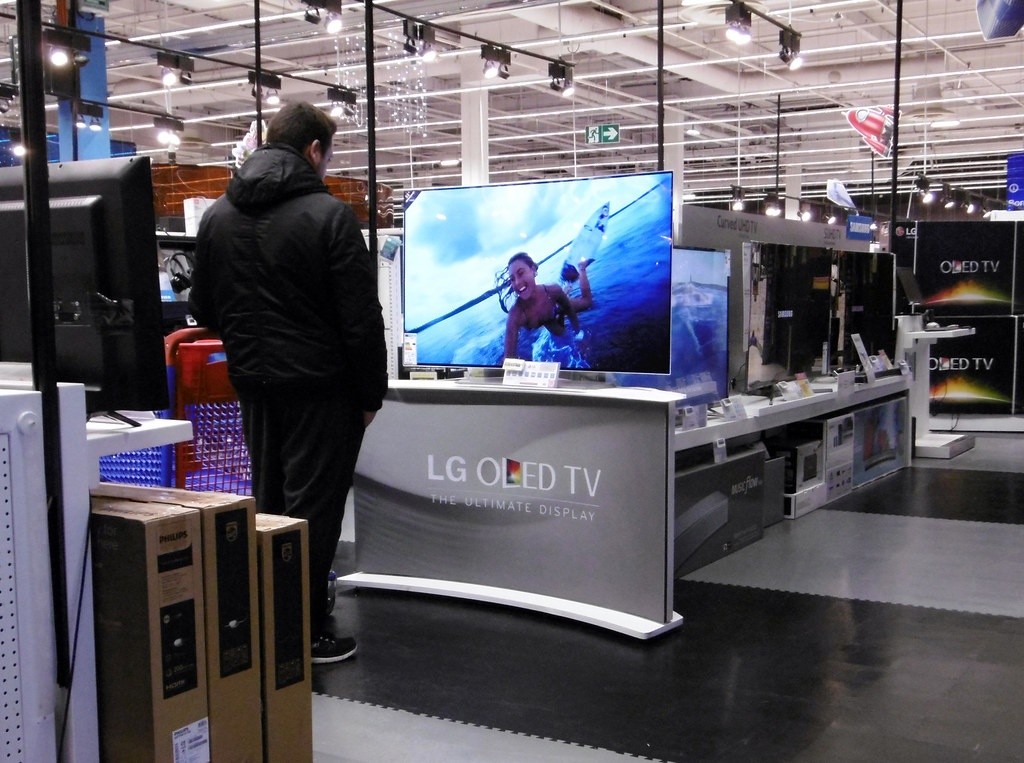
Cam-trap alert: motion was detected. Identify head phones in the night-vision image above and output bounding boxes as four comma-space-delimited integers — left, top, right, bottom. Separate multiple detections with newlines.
170, 253, 196, 293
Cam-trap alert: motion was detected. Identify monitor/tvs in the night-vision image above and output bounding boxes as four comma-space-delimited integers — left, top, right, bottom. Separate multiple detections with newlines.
0, 156, 170, 419
746, 220, 1024, 418
402, 170, 731, 408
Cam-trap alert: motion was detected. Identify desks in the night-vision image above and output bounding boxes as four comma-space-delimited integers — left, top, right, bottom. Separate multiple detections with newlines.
905, 328, 976, 459
674, 371, 913, 467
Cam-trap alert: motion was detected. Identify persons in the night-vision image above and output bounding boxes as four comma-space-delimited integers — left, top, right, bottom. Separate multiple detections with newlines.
186, 101, 388, 663
495, 253, 595, 359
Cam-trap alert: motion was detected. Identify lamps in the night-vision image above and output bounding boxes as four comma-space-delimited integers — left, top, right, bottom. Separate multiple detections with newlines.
920, 190, 933, 204
979, 206, 991, 219
764, 202, 776, 215
420, 48, 438, 62
482, 64, 499, 80
779, 49, 803, 71
797, 209, 812, 222
960, 201, 975, 215
49, 47, 344, 119
824, 214, 837, 225
941, 196, 955, 208
551, 81, 574, 98
324, 17, 342, 35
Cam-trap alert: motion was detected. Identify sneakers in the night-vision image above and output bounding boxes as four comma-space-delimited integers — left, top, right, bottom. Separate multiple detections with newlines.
311, 634, 359, 663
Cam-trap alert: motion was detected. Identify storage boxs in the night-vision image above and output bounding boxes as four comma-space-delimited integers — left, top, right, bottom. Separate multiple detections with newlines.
891, 219, 1024, 416
88, 497, 211, 763
256, 512, 314, 763
89, 481, 265, 763
674, 395, 906, 580
